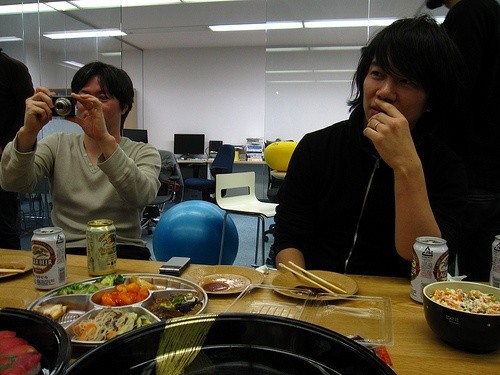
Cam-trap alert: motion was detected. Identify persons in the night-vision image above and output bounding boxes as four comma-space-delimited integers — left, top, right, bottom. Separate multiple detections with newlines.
0, 62, 162, 260
272, 0, 500, 281
0, 48, 35, 250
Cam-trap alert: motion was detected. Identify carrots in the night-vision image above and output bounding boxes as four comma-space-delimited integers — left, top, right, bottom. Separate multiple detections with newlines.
73, 323, 116, 341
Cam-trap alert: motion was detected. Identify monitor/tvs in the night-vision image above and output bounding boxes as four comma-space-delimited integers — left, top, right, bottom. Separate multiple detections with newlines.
174, 134, 205, 155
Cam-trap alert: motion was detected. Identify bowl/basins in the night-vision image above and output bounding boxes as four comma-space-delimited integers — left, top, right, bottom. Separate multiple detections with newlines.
60, 312, 398, 375
0, 307, 71, 375
423, 281, 500, 354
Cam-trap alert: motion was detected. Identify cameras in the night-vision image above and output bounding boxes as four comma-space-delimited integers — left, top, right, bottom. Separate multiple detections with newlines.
49, 97, 76, 117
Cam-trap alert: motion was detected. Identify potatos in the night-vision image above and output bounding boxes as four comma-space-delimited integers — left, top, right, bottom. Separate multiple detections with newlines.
94, 283, 150, 306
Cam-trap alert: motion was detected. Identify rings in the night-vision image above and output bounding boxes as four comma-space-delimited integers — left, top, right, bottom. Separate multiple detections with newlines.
374, 122, 382, 130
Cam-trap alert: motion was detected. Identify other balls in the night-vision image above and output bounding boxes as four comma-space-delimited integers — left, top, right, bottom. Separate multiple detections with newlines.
152, 199, 240, 265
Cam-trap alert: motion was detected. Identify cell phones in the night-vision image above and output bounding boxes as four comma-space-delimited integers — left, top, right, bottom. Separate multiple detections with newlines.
159, 257, 191, 277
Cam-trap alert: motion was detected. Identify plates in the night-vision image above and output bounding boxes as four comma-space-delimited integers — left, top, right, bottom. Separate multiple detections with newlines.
0, 261, 32, 278
272, 270, 358, 300
181, 266, 264, 294
27, 272, 208, 346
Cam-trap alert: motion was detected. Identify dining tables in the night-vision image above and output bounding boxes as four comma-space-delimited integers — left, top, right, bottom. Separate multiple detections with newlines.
0, 249, 500, 375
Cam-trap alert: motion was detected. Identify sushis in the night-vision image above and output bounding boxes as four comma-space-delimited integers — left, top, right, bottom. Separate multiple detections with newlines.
0, 330, 42, 375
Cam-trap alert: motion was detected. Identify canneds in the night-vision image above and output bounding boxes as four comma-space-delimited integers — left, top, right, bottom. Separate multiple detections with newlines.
86, 219, 118, 276
490, 235, 500, 289
31, 227, 67, 290
409, 235, 449, 304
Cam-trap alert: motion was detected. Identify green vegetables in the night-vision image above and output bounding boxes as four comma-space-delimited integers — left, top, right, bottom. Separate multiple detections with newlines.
157, 294, 186, 308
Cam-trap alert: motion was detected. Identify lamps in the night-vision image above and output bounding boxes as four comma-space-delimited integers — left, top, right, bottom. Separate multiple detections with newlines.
209, 18, 400, 33
42, 27, 127, 40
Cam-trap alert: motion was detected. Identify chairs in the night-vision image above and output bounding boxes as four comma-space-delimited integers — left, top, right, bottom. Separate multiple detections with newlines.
145, 141, 298, 266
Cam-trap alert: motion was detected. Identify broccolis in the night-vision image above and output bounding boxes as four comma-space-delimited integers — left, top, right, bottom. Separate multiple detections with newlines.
58, 273, 126, 296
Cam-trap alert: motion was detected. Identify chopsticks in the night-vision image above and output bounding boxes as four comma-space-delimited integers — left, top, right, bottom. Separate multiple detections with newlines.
279, 261, 348, 297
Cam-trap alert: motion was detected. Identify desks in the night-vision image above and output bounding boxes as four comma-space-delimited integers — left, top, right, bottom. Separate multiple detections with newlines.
176, 157, 208, 201
207, 158, 268, 202
